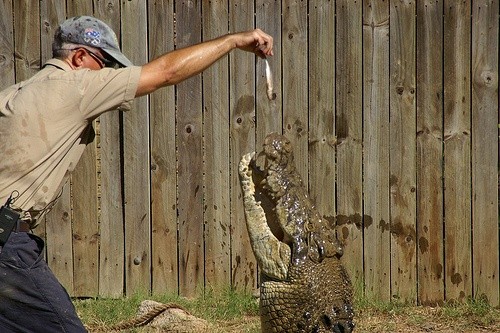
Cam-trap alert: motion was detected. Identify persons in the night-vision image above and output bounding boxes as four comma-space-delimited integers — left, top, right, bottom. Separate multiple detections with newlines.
0, 15, 275, 333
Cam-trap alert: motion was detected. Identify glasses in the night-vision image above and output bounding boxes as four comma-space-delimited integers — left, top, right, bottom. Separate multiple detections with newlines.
74, 47, 116, 68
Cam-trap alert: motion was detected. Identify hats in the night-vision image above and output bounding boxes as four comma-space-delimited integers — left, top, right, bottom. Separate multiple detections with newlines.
52, 15, 135, 67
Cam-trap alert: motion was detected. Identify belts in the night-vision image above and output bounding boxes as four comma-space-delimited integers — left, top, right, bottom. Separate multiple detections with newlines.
13, 221, 30, 233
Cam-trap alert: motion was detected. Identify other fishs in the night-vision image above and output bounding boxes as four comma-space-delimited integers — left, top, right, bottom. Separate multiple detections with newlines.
264, 57, 274, 100
